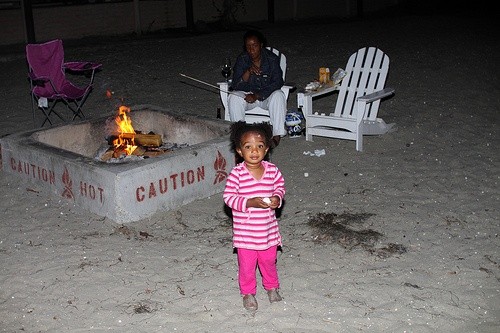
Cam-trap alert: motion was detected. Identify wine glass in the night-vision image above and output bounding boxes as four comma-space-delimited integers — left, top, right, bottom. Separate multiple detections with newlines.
222, 65, 232, 83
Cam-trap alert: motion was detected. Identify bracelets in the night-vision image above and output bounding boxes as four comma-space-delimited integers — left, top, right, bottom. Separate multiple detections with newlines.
247, 67, 253, 75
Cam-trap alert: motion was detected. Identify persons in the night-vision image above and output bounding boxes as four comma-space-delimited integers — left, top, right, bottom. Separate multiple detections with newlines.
224, 120, 286, 312
220, 30, 289, 151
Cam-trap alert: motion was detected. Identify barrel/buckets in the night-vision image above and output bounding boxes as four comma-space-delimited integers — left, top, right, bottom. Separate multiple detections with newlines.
285, 112, 302, 138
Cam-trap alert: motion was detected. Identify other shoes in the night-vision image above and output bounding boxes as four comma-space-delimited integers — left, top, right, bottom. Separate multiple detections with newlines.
271, 135, 280, 148
268, 288, 282, 303
242, 295, 258, 310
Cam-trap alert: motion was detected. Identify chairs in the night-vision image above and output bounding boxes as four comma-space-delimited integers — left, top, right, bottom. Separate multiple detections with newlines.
217, 47, 293, 124
26, 39, 102, 128
297, 47, 396, 152
